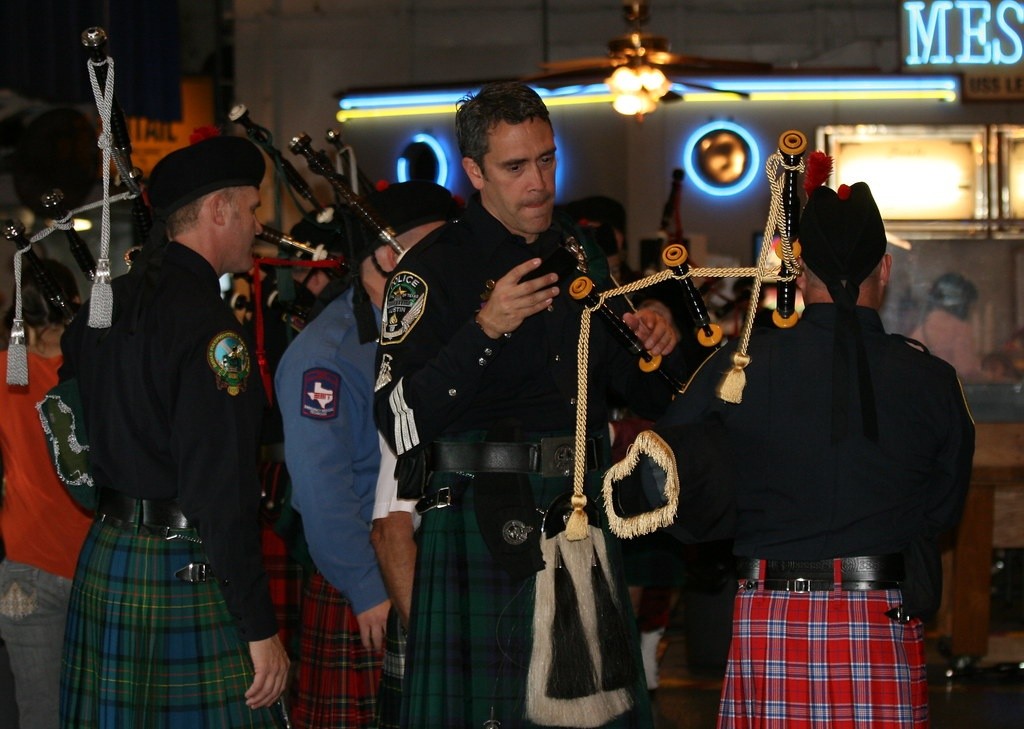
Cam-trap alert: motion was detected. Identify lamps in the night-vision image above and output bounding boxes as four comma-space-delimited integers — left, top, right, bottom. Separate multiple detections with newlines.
606, 65, 671, 115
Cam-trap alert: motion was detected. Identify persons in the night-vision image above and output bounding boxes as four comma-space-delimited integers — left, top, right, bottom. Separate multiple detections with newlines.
1, 80, 975, 729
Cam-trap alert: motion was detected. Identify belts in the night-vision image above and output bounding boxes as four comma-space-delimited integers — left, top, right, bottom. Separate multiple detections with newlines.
89, 486, 198, 531
738, 556, 907, 590
427, 434, 607, 477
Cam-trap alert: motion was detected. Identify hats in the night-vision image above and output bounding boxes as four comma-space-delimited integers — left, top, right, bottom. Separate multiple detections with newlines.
287, 202, 363, 258
145, 123, 266, 222
552, 195, 626, 258
799, 181, 887, 285
340, 179, 460, 258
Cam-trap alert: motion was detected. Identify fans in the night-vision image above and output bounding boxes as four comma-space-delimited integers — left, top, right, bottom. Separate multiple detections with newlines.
523, 0, 751, 105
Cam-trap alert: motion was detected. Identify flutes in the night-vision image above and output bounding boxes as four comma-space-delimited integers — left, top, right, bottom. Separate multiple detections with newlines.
568, 129, 809, 396
226, 103, 407, 284
1, 23, 157, 330
